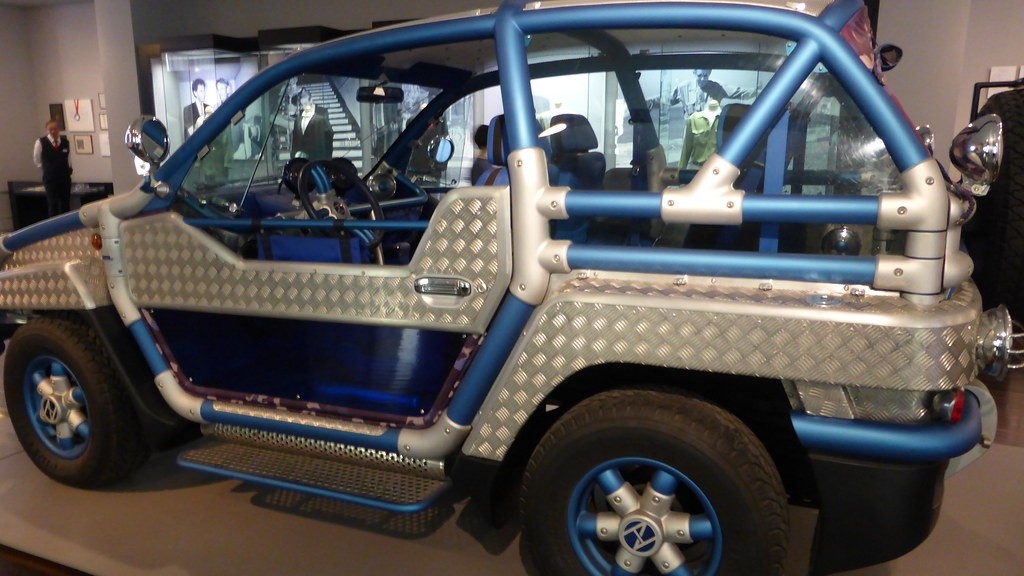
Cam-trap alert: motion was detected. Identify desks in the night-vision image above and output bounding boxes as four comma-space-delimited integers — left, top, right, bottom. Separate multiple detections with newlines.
17, 186, 106, 231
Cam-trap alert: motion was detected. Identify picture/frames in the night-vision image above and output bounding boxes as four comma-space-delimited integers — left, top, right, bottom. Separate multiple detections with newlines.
73, 134, 94, 156
49, 103, 65, 131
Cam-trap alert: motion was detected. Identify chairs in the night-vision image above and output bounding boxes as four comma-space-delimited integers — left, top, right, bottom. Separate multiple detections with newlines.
681, 104, 811, 252
483, 113, 608, 247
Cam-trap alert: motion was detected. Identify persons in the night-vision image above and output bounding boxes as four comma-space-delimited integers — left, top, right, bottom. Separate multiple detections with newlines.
645, 69, 763, 112
294, 90, 329, 122
184, 78, 210, 142
33, 119, 73, 217
290, 105, 334, 160
213, 77, 244, 154
678, 99, 722, 170
471, 124, 493, 186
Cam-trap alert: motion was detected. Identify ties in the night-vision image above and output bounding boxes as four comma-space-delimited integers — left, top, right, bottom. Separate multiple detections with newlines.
54, 140, 58, 151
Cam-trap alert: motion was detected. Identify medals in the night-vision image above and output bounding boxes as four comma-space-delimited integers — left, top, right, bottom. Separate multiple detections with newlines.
74, 113, 80, 121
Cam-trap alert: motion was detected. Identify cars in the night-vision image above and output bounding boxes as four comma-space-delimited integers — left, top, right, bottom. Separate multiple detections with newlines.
2, 0, 1024, 576
